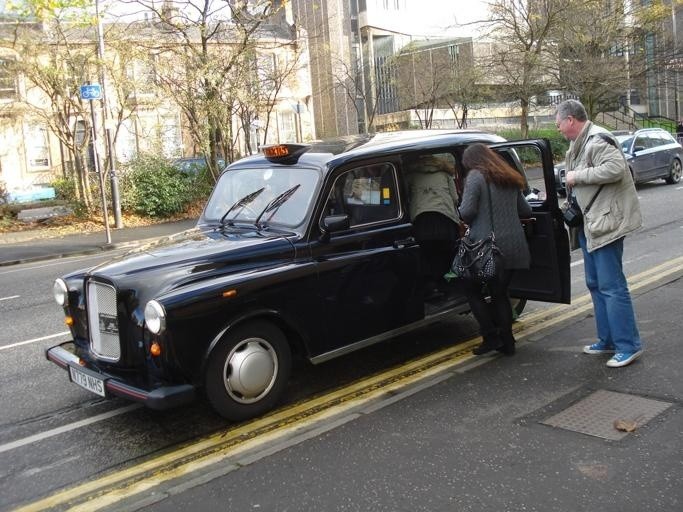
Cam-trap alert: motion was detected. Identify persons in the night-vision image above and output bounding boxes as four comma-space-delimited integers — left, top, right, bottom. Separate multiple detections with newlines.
456, 140, 530, 356
551, 97, 645, 371
408, 149, 462, 306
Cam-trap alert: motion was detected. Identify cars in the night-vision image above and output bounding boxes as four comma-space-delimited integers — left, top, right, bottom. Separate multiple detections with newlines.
554, 127, 683, 198
43, 128, 573, 424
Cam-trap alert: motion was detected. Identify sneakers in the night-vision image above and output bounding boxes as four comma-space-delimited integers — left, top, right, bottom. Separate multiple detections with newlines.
605, 349, 644, 369
425, 283, 446, 303
581, 340, 615, 355
471, 335, 517, 356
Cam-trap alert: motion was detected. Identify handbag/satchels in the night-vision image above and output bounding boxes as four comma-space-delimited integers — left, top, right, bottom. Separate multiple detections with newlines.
448, 234, 505, 283
